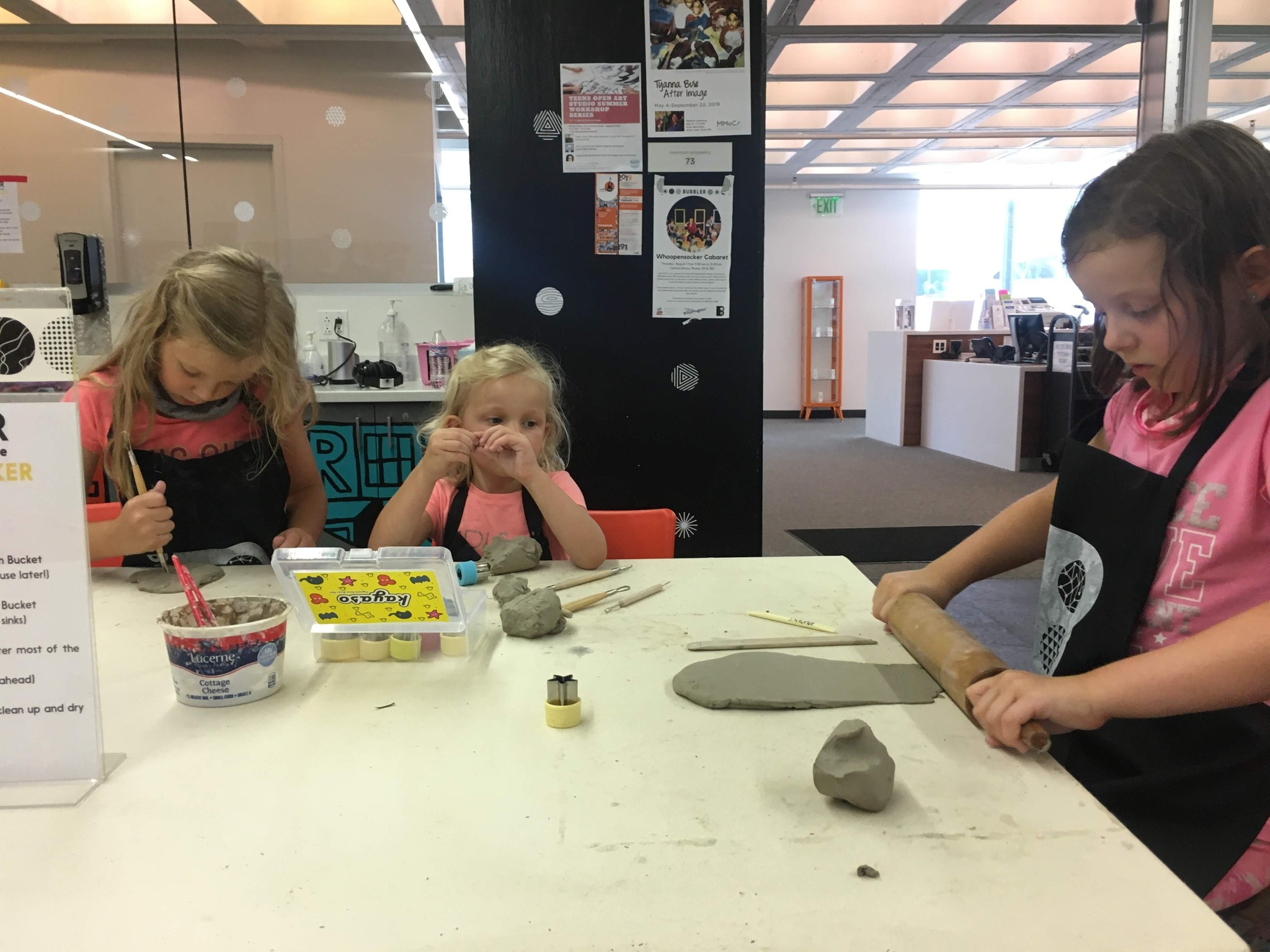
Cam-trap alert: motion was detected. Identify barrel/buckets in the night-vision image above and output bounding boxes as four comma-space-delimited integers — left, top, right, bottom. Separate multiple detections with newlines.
415, 339, 476, 384
157, 596, 292, 708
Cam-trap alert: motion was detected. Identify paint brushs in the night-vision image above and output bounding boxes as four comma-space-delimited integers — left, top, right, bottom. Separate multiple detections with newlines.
121, 431, 168, 572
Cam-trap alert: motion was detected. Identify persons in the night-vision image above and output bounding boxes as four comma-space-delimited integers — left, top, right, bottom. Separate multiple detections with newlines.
367, 343, 607, 570
61, 243, 328, 562
873, 118, 1270, 952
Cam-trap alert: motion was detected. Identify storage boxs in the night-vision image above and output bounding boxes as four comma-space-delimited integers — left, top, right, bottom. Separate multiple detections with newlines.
270, 546, 496, 662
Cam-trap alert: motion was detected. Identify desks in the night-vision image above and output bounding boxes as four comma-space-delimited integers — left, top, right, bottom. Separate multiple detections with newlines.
0, 554, 1251, 952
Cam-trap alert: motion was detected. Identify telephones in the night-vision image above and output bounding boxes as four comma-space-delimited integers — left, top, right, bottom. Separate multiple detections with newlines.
971, 337, 997, 359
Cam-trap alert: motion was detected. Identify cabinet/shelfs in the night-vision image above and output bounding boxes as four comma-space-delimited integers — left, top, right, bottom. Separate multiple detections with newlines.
801, 274, 846, 421
302, 402, 444, 542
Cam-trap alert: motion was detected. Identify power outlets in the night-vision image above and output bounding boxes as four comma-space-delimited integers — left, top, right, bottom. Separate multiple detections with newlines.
319, 310, 348, 341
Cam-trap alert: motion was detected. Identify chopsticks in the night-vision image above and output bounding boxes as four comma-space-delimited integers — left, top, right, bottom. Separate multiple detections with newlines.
171, 554, 219, 627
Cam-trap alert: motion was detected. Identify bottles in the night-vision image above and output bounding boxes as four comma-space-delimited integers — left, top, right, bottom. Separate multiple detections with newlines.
429, 330, 450, 389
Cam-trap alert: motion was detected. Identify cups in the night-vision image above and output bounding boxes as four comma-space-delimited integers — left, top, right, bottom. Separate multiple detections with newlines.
815, 327, 820, 336
830, 298, 835, 308
830, 369, 835, 379
818, 392, 823, 401
813, 369, 818, 379
827, 327, 833, 337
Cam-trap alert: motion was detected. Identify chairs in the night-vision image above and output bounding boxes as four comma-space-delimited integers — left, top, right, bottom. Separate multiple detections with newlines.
583, 508, 677, 561
